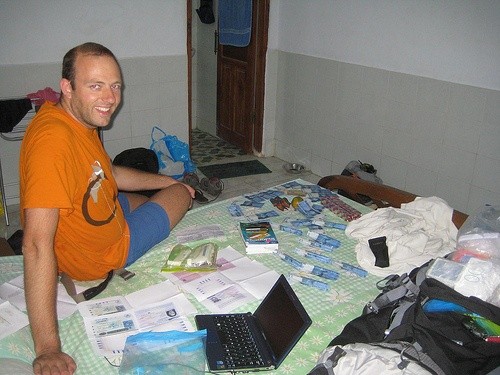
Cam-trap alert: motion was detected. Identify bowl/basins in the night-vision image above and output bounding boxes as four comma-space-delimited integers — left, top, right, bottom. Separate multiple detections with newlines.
284, 163, 305, 174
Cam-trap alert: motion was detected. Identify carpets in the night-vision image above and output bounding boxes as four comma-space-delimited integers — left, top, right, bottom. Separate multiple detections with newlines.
197, 159, 272, 180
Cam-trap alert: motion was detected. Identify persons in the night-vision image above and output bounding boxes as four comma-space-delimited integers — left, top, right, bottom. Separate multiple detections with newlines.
21, 42, 194, 375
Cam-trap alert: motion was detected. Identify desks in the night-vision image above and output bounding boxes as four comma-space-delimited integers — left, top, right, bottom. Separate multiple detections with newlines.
0, 99, 104, 226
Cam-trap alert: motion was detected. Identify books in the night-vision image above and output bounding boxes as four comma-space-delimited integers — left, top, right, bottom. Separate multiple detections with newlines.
238, 219, 279, 257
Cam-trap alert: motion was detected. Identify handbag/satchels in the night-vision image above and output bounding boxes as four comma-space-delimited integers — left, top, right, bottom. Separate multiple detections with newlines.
150, 126, 194, 179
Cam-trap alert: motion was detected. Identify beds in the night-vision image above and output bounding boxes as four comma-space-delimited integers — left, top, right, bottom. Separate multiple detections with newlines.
0, 176, 472, 375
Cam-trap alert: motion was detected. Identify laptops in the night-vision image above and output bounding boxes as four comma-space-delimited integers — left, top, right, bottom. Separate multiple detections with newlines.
194, 274, 312, 373
